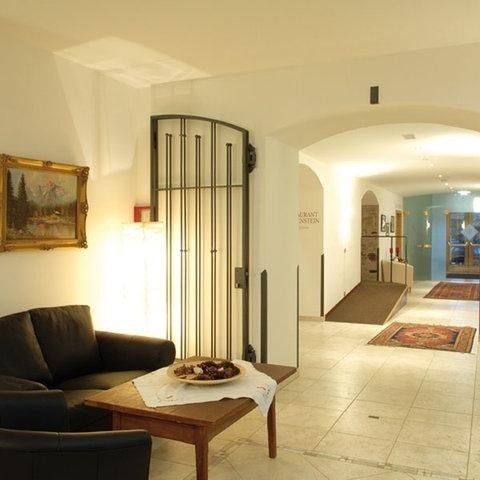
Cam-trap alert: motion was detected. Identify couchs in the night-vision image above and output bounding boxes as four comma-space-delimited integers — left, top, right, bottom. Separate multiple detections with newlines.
0, 304, 176, 432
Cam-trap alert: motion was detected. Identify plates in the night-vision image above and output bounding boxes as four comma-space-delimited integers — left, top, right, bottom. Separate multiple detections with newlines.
167, 360, 247, 386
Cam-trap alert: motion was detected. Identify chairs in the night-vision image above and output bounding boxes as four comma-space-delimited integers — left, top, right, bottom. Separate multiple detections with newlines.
1, 389, 153, 480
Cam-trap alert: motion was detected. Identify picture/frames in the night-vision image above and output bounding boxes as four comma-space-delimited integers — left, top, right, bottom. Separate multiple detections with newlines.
0, 153, 90, 252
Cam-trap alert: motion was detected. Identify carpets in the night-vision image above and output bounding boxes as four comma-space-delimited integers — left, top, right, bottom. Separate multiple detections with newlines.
423, 281, 480, 302
367, 321, 476, 352
